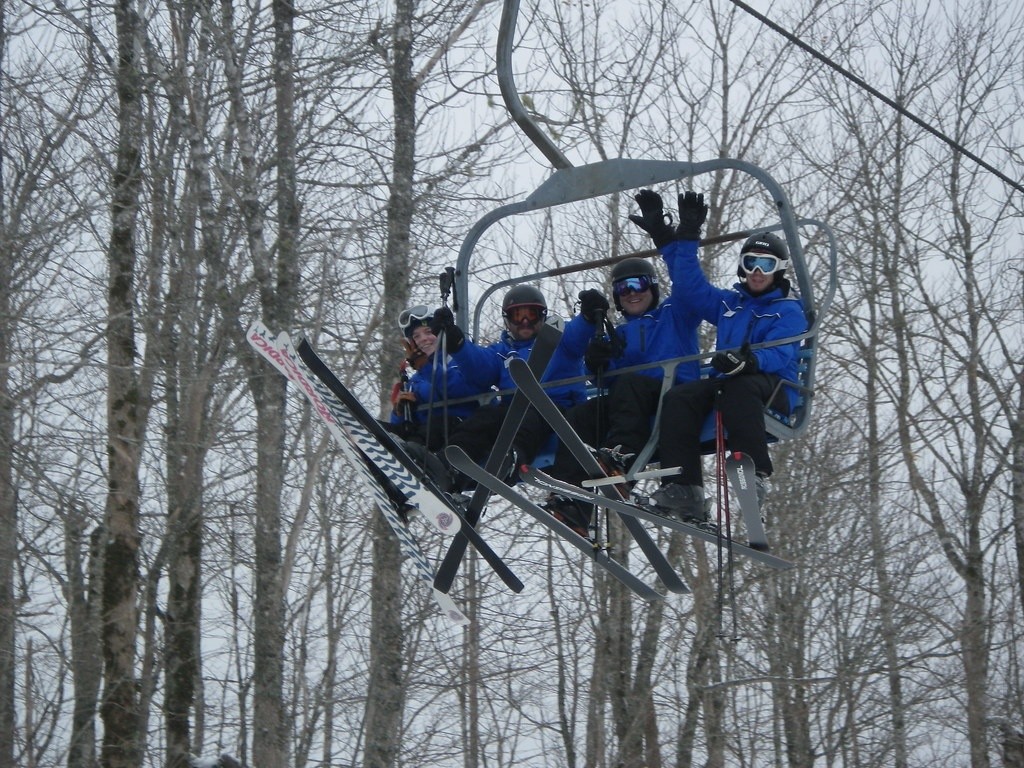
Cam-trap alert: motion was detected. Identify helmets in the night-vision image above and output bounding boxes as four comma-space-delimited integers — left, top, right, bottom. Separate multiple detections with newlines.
612, 258, 660, 310
502, 284, 548, 322
737, 231, 788, 279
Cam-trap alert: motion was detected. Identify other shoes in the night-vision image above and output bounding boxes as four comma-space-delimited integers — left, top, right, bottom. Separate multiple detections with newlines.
444, 490, 473, 513
480, 444, 517, 481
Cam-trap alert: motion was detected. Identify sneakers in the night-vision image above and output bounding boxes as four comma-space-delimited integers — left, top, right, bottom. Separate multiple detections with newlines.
387, 487, 424, 517
535, 495, 591, 540
407, 441, 467, 501
584, 443, 637, 500
653, 481, 708, 522
375, 418, 427, 453
755, 469, 769, 488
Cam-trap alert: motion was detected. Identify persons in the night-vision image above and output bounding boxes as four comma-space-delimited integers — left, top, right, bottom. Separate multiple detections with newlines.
636, 190, 809, 529
538, 189, 703, 537
404, 285, 609, 495
383, 307, 497, 453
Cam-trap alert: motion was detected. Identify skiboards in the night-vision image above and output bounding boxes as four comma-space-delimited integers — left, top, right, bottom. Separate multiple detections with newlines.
243, 317, 799, 629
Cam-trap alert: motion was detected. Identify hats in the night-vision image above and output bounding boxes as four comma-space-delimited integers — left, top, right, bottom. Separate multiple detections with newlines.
402, 304, 440, 346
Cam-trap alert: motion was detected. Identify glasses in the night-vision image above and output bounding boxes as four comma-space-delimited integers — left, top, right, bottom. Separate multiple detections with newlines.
612, 275, 659, 295
503, 306, 546, 326
738, 252, 790, 275
398, 305, 436, 334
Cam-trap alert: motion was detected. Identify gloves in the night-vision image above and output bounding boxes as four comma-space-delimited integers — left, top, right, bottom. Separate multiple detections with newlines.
578, 288, 610, 324
396, 389, 419, 420
712, 338, 761, 384
629, 188, 677, 252
671, 191, 709, 242
432, 307, 466, 356
401, 337, 430, 370
584, 338, 615, 373
